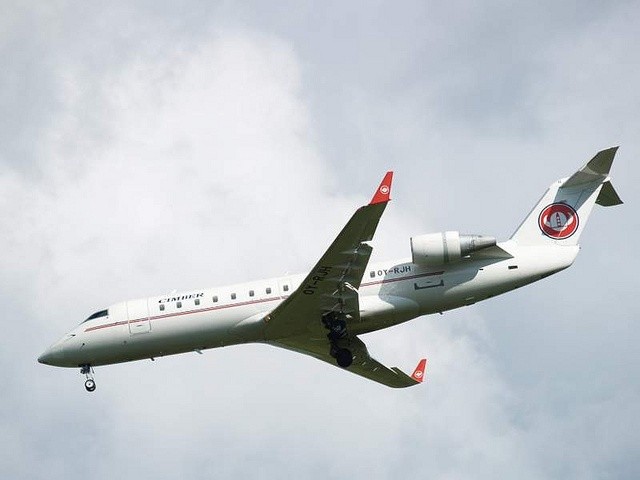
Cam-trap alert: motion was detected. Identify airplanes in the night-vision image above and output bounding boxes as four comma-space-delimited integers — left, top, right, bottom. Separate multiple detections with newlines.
38, 146, 623, 389
